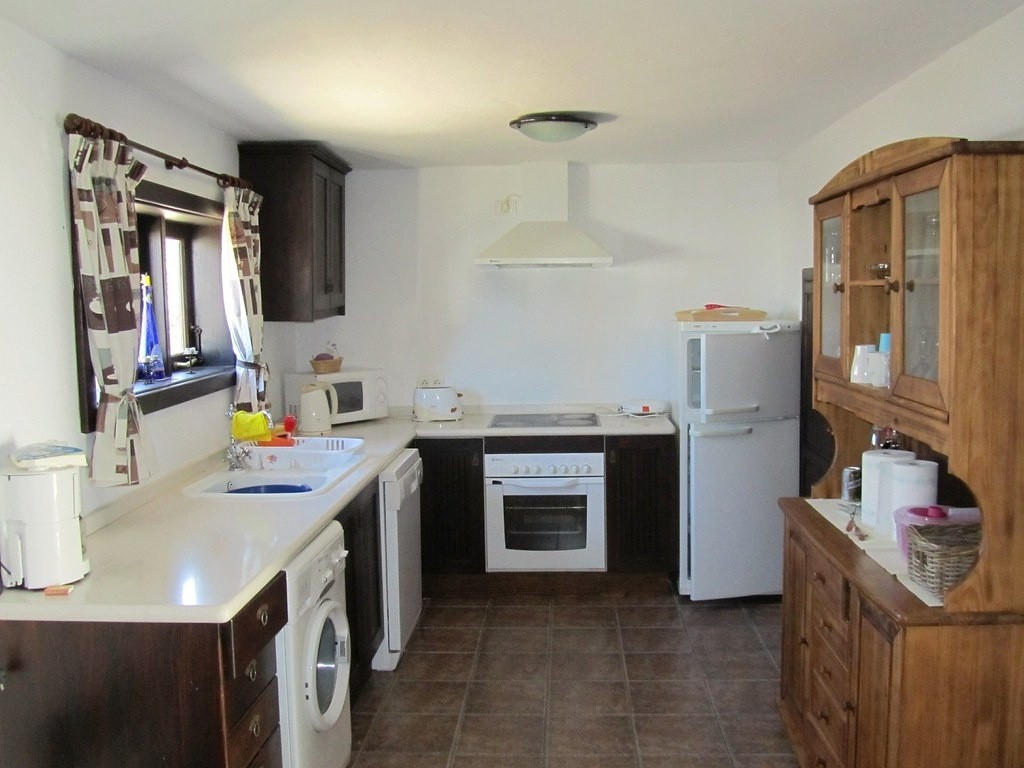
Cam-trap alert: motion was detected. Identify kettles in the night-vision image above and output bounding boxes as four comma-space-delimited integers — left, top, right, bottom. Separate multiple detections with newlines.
296, 381, 338, 435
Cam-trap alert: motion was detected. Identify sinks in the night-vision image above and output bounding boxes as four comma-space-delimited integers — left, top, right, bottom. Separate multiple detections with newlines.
196, 473, 328, 494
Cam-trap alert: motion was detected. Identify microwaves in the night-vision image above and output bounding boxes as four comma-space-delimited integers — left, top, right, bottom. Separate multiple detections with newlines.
283, 367, 388, 424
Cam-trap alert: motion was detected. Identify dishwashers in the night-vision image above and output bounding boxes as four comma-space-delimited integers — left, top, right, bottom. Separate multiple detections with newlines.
378, 448, 423, 650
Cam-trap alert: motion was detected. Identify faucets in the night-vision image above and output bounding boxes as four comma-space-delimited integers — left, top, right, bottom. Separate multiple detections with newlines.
225, 400, 275, 471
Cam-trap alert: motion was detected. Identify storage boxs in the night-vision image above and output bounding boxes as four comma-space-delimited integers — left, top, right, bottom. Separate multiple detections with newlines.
235, 436, 364, 472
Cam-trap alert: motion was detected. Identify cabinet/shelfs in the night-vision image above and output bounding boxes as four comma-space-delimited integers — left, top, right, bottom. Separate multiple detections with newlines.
0, 570, 290, 768
799, 135, 1024, 613
605, 436, 678, 574
775, 495, 1024, 768
237, 142, 354, 322
337, 476, 384, 702
412, 439, 485, 575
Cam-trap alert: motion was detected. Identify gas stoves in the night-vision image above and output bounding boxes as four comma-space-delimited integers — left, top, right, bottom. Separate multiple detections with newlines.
487, 413, 600, 428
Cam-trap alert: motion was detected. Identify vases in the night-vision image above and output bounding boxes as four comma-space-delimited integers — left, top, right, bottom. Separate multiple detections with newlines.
138, 285, 167, 380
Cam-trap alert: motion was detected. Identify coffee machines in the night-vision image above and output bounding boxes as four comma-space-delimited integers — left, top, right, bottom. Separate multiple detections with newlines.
0, 464, 91, 590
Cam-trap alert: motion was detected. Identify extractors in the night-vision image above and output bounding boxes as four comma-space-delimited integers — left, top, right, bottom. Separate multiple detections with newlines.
475, 160, 613, 268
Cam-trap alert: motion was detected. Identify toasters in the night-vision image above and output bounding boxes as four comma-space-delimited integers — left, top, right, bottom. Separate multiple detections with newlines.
411, 387, 464, 422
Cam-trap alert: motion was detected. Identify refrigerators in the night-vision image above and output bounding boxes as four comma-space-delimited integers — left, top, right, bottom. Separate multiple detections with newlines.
674, 321, 802, 601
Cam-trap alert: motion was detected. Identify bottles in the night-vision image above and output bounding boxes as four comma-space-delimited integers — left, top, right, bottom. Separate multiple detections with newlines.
151, 355, 165, 379
137, 286, 163, 379
143, 355, 154, 385
867, 422, 904, 450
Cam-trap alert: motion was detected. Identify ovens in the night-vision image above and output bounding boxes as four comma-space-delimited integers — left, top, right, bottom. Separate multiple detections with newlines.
483, 453, 607, 572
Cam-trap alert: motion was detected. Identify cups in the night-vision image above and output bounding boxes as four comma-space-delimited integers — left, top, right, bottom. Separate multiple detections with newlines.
867, 351, 889, 387
879, 333, 890, 352
850, 345, 877, 383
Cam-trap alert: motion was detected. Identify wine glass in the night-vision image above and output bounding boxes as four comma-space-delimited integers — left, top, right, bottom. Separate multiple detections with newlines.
909, 327, 938, 380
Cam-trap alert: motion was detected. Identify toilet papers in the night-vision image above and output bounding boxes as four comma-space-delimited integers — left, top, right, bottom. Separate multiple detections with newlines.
890, 460, 939, 543
860, 449, 914, 529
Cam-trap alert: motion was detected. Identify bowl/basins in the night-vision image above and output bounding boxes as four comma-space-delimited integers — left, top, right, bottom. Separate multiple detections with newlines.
893, 505, 960, 561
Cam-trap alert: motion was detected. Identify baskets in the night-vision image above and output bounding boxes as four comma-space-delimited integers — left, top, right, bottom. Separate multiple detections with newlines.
908, 522, 979, 601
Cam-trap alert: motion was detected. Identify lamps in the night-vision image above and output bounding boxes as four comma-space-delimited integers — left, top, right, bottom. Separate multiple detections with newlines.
510, 113, 599, 146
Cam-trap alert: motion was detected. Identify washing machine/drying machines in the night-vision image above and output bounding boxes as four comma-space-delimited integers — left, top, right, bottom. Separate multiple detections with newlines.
271, 517, 352, 768
